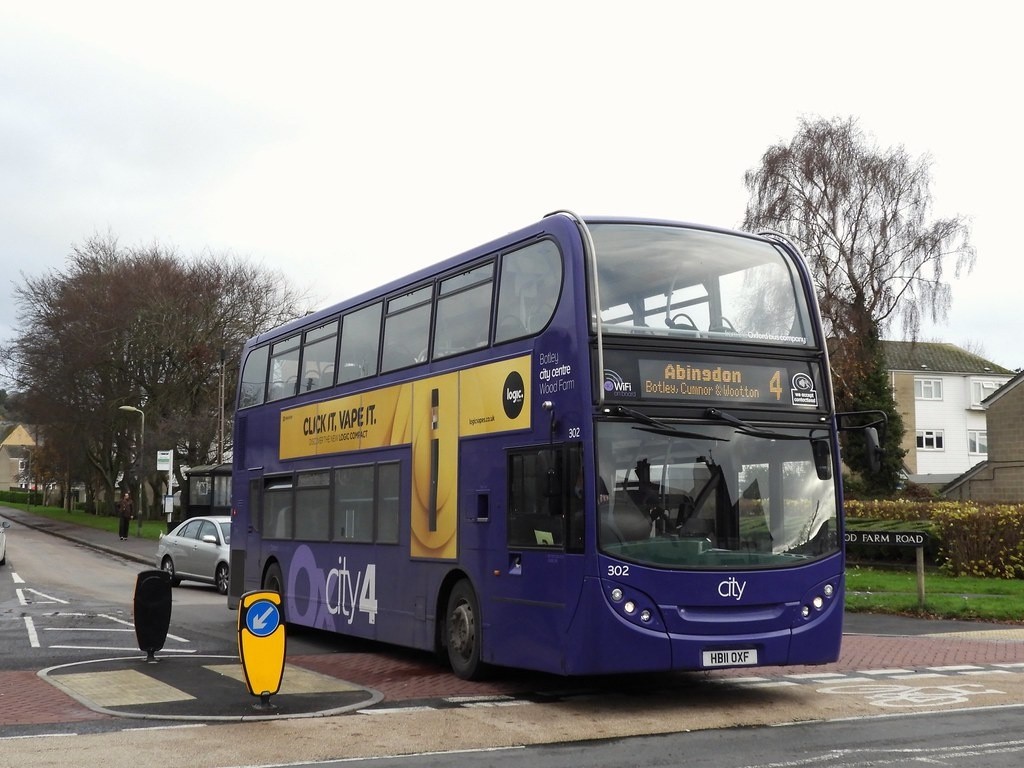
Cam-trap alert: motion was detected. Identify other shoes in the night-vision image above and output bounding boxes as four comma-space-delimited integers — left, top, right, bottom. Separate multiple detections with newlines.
120, 537, 127, 540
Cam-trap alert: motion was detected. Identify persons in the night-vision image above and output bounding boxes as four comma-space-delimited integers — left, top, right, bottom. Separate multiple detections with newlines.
546, 453, 611, 519
117, 493, 134, 540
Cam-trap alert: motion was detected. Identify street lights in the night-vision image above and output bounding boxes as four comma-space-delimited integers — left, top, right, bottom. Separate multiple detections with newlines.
121, 405, 145, 536
21, 447, 31, 509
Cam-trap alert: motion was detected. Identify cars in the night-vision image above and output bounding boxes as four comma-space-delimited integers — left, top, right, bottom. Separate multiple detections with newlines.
156, 515, 232, 594
0, 521, 10, 564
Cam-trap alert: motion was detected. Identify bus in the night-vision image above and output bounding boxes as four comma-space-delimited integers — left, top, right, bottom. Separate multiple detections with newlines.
228, 209, 888, 683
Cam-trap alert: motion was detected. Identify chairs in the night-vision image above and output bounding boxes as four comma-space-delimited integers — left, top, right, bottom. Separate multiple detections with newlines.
667, 313, 704, 338
708, 318, 737, 338
251, 304, 551, 405
631, 324, 655, 336
